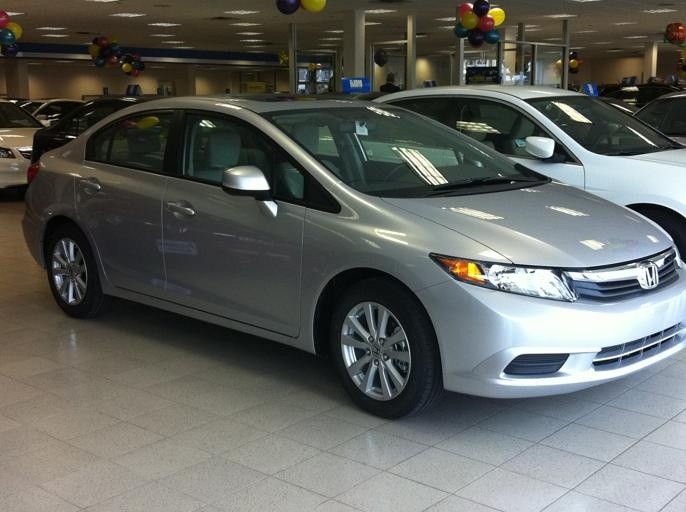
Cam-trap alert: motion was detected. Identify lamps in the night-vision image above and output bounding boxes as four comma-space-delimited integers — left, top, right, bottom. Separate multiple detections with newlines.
126, 132, 162, 169
465, 115, 541, 162
195, 126, 343, 201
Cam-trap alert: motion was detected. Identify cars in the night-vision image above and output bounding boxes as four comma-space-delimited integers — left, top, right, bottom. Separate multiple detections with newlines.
316, 84, 684, 264
626, 92, 685, 143
22, 94, 685, 422
1, 96, 89, 203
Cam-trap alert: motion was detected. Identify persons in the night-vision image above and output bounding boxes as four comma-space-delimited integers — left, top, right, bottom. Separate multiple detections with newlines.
380, 72, 400, 92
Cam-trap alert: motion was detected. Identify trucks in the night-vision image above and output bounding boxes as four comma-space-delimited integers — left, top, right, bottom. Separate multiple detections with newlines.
0, 11, 23, 59
88, 36, 145, 76
276, 0, 300, 14
664, 23, 686, 47
300, 0, 327, 14
120, 116, 167, 149
556, 51, 583, 74
454, 0, 505, 47
